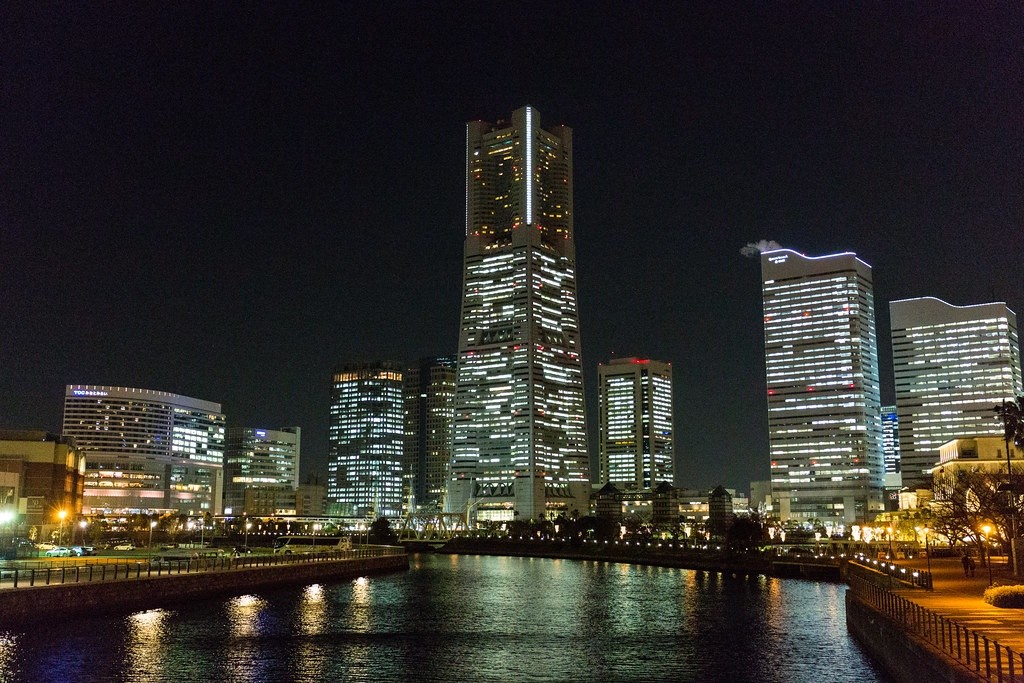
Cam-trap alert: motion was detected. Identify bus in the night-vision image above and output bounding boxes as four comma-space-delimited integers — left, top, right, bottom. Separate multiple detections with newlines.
272, 534, 353, 557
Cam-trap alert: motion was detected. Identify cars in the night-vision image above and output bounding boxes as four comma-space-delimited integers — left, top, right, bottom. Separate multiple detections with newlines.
231, 545, 254, 556
21, 539, 93, 558
114, 542, 136, 552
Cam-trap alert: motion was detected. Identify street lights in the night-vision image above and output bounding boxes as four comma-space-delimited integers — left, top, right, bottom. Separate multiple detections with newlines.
148, 519, 157, 555
887, 527, 892, 563
924, 523, 931, 574
58, 510, 67, 547
981, 526, 992, 585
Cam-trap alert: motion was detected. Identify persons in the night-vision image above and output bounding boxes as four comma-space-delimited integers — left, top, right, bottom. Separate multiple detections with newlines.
961, 553, 970, 577
969, 557, 975, 577
231, 548, 240, 557
